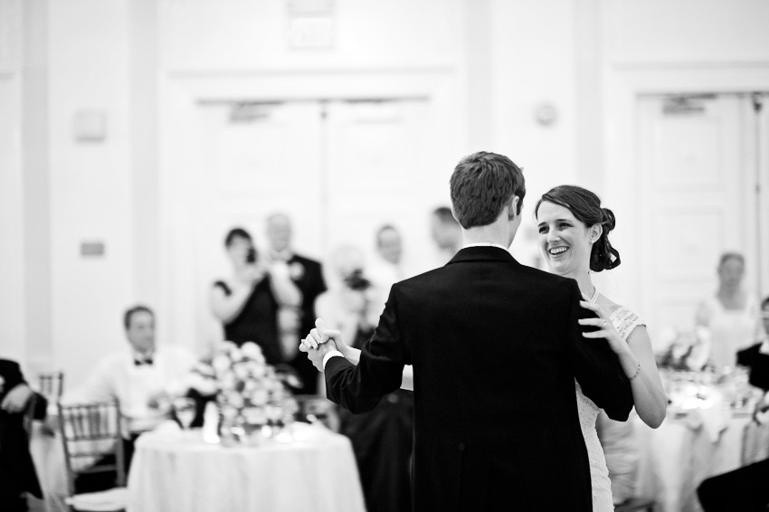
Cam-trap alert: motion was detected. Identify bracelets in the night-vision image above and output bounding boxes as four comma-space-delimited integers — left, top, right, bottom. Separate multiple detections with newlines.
628, 363, 641, 380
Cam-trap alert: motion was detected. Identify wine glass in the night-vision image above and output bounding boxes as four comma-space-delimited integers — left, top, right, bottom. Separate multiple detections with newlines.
173, 399, 197, 433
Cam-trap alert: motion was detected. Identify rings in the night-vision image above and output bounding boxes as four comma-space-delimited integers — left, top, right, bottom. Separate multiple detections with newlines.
602, 318, 608, 328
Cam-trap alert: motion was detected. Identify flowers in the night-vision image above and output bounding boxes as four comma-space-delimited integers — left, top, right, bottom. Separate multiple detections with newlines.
205, 340, 297, 445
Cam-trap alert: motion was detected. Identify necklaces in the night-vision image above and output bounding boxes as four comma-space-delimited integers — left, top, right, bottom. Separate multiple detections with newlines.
589, 287, 599, 305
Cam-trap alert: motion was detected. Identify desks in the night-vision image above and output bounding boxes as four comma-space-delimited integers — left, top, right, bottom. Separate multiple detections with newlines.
127, 415, 364, 512
603, 367, 768, 512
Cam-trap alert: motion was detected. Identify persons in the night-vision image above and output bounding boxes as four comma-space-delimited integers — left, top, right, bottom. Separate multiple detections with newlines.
298, 151, 635, 512
209, 212, 328, 394
301, 185, 668, 512
696, 254, 769, 512
0, 355, 49, 512
113, 309, 193, 439
315, 208, 459, 432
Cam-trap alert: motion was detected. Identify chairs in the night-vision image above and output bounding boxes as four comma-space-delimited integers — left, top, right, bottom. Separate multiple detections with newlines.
0, 372, 125, 512
